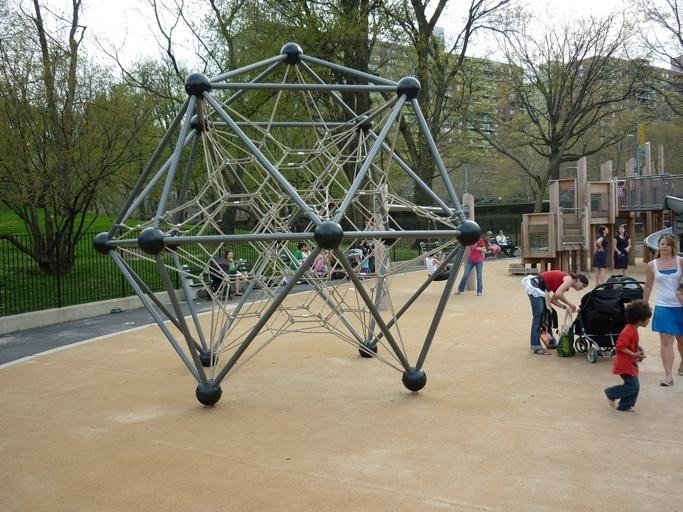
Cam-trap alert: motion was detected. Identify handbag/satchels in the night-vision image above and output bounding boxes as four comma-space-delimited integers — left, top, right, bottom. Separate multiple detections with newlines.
540, 330, 552, 346
676, 286, 683, 306
555, 330, 576, 357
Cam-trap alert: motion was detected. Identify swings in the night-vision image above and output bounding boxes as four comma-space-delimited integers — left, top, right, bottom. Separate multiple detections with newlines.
420, 221, 454, 281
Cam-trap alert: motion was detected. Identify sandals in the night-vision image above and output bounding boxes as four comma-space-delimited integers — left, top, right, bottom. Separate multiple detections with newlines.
659, 378, 673, 386
534, 349, 552, 355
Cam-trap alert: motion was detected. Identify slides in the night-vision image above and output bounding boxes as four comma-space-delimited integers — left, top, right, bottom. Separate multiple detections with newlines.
645, 196, 683, 287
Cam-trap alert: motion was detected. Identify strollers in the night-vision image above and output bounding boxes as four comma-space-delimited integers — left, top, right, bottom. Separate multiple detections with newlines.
567, 274, 646, 363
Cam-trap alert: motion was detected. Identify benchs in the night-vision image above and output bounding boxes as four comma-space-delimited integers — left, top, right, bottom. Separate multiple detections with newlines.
484, 237, 518, 259
418, 241, 457, 265
278, 254, 304, 284
180, 258, 265, 301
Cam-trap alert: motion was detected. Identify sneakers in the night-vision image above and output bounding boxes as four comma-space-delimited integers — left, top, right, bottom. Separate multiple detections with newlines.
678, 368, 683, 376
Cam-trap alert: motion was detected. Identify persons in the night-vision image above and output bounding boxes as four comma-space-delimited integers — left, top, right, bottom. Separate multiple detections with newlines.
521, 269, 588, 355
642, 234, 683, 386
224, 251, 250, 296
290, 218, 375, 284
425, 229, 516, 295
604, 299, 652, 412
592, 224, 632, 285
